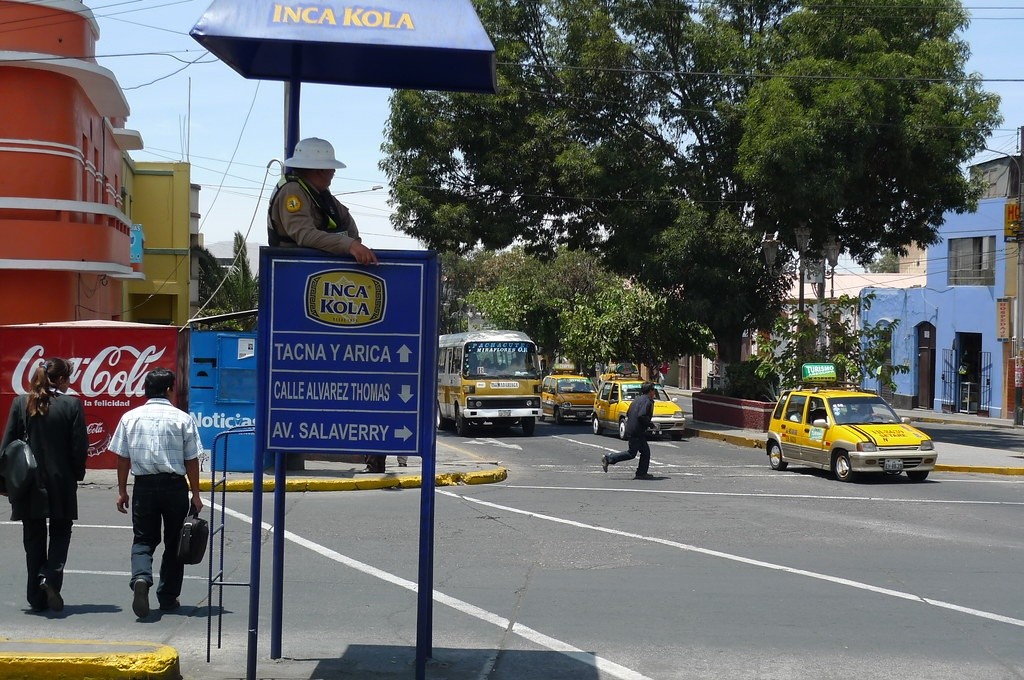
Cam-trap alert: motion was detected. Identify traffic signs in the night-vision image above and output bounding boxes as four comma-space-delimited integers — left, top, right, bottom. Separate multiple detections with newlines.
269, 413, 422, 456
268, 331, 420, 372
269, 374, 419, 412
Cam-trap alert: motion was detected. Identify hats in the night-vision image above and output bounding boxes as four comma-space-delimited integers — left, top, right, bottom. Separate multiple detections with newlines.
798, 398, 805, 406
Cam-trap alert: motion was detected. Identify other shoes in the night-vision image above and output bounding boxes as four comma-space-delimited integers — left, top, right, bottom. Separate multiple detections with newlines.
636, 472, 653, 479
160, 597, 180, 611
30, 600, 48, 612
39, 577, 64, 611
132, 579, 149, 619
601, 454, 610, 473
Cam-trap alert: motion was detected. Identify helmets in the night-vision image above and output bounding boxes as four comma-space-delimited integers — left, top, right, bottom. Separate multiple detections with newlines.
284, 137, 346, 168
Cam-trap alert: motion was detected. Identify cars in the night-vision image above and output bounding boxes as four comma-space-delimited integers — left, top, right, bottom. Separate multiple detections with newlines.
591, 363, 686, 443
542, 364, 599, 425
773, 362, 938, 484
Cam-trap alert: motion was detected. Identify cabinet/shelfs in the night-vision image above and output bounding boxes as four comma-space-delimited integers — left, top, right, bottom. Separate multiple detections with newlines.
960, 382, 978, 414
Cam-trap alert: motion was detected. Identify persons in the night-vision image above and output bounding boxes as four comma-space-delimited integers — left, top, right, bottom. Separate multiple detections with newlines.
108, 368, 203, 618
511, 358, 521, 368
362, 455, 387, 472
850, 404, 875, 421
550, 367, 558, 375
0, 356, 89, 615
602, 381, 656, 479
483, 358, 496, 370
268, 137, 380, 266
659, 361, 670, 386
790, 398, 812, 424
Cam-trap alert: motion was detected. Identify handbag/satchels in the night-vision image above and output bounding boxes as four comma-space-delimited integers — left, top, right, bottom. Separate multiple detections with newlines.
0, 394, 39, 494
178, 500, 209, 564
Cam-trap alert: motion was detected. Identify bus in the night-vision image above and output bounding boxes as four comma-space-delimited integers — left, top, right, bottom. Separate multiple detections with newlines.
439, 330, 544, 436
